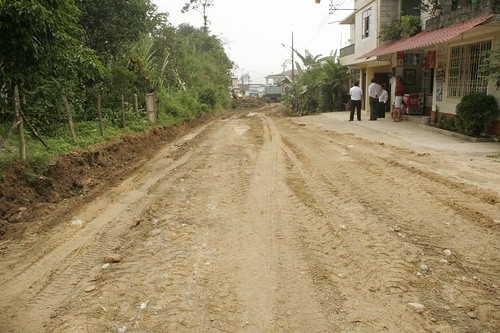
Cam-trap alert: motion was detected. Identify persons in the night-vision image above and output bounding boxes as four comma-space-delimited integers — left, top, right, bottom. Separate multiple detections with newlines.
369, 78, 381, 122
347, 81, 362, 121
390, 73, 402, 123
377, 84, 388, 118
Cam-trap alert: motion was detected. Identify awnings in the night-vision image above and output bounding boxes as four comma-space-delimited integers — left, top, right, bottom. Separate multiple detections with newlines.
355, 11, 495, 61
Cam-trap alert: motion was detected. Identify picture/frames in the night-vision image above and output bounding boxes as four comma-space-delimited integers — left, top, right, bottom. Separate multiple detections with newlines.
403, 68, 416, 87
